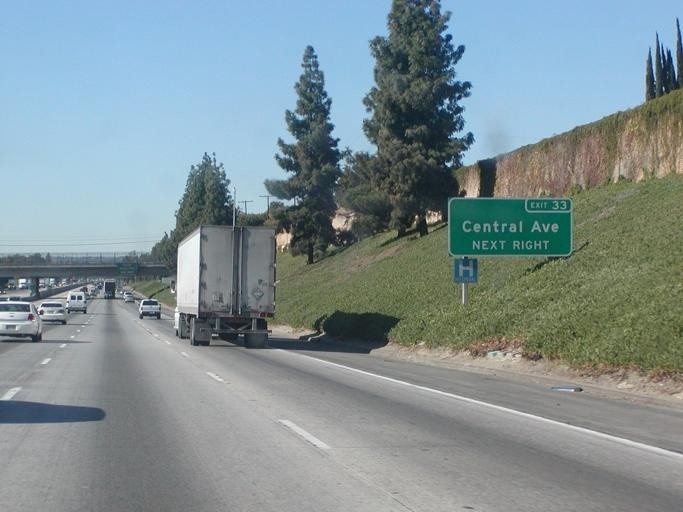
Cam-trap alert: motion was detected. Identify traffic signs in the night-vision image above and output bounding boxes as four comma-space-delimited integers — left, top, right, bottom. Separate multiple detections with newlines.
447, 197, 573, 259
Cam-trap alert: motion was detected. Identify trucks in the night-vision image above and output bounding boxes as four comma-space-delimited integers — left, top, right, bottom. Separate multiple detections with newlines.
170, 222, 279, 350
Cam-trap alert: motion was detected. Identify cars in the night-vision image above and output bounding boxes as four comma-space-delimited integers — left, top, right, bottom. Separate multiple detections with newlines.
34, 300, 68, 326
0, 300, 43, 343
0, 277, 137, 315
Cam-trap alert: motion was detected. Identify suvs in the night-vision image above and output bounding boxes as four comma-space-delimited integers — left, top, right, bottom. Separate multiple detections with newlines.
138, 296, 161, 320
